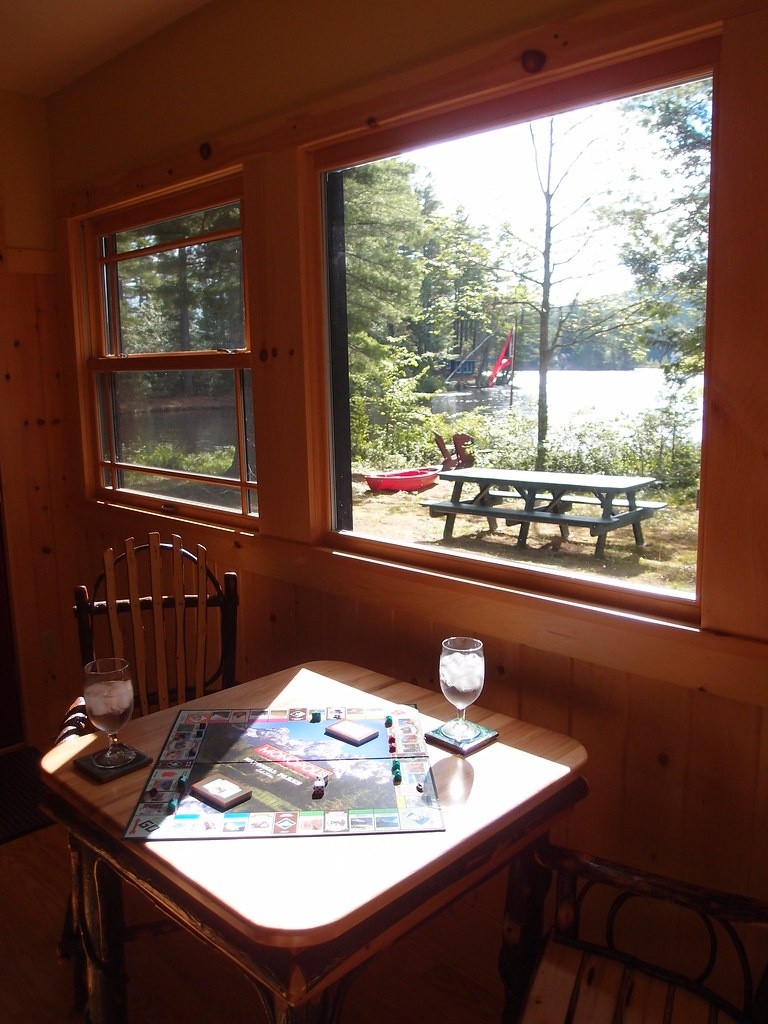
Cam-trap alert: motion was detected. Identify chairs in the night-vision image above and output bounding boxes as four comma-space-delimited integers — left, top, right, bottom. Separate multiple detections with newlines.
501, 843, 768, 1024
56, 529, 240, 1013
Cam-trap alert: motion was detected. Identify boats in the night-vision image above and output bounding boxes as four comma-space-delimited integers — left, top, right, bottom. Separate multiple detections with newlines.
366, 465, 442, 492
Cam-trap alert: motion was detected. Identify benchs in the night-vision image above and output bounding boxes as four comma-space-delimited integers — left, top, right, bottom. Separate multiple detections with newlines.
477, 490, 669, 545
419, 501, 621, 561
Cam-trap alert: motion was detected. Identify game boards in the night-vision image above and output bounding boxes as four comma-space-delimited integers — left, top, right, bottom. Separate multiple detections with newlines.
121, 703, 446, 839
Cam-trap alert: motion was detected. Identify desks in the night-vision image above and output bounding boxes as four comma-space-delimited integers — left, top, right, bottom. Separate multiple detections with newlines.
38, 659, 593, 1024
436, 467, 656, 558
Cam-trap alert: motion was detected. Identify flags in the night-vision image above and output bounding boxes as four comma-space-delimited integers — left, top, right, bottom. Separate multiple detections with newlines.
489, 329, 513, 387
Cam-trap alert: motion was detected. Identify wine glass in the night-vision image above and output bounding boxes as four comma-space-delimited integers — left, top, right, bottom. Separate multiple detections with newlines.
83, 659, 137, 769
439, 636, 485, 740
